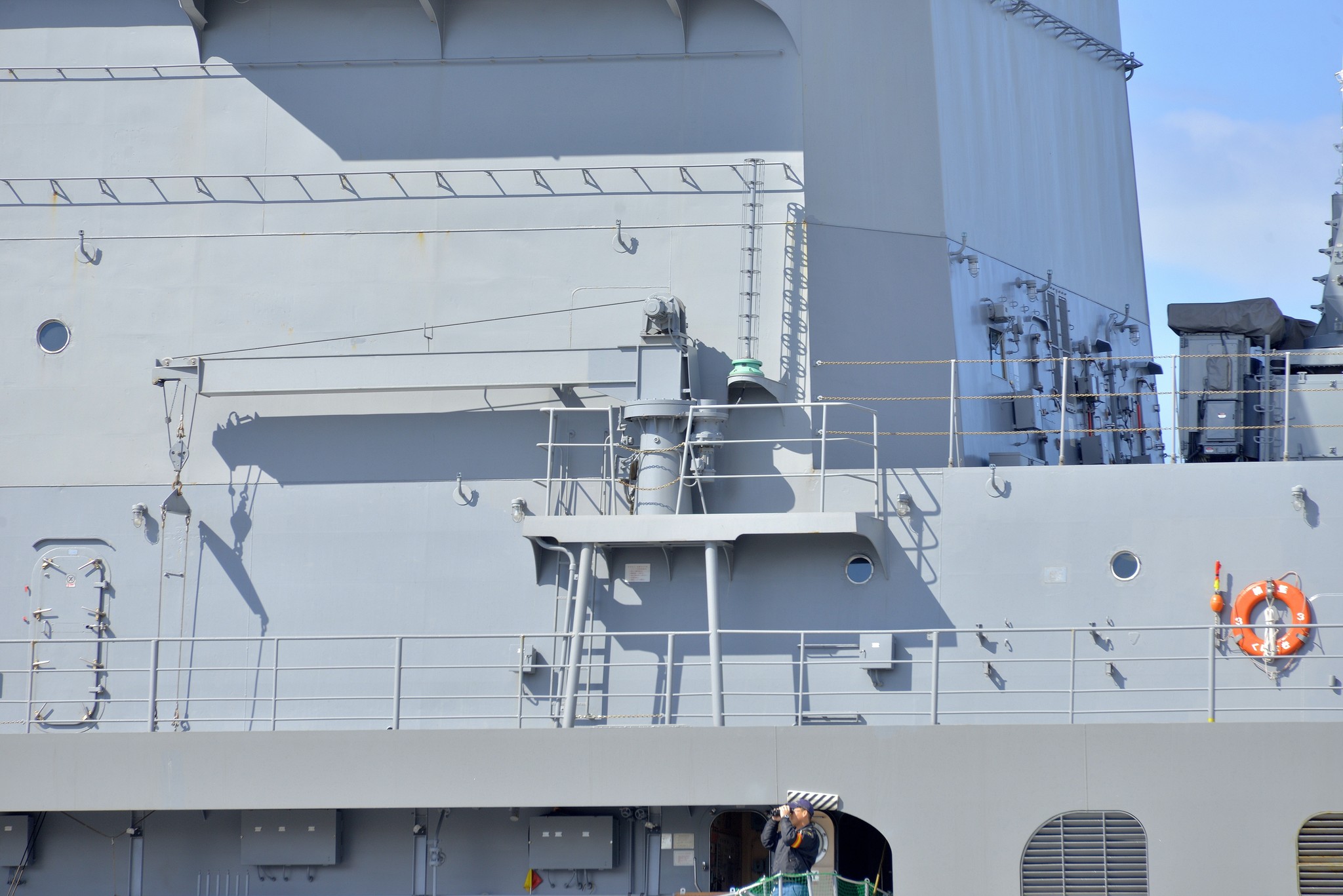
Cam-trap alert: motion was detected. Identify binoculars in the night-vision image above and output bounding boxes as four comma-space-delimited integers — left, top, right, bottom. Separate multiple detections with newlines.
770, 808, 793, 817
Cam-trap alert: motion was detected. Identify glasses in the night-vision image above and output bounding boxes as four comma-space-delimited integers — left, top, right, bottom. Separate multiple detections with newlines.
791, 809, 804, 814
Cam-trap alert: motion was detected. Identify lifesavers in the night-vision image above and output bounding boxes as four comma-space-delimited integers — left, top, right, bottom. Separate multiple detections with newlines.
1230, 579, 1312, 658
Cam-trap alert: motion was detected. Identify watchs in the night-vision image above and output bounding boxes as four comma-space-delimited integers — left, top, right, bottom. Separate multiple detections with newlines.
782, 814, 789, 820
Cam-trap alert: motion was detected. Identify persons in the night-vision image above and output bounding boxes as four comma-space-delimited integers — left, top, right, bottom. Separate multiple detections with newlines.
760, 799, 820, 896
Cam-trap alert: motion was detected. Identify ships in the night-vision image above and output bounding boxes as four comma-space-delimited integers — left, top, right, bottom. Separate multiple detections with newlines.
1, 2, 1340, 896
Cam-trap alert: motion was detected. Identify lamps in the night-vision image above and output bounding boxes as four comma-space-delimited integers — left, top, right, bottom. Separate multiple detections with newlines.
130, 502, 148, 529
959, 252, 980, 278
510, 496, 526, 523
1017, 276, 1039, 302
1291, 484, 1305, 512
1115, 323, 1140, 346
895, 491, 913, 518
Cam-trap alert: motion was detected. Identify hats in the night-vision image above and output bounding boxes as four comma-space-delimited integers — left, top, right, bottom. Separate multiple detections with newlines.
785, 799, 814, 816
